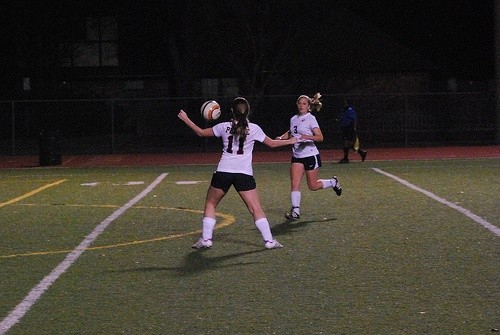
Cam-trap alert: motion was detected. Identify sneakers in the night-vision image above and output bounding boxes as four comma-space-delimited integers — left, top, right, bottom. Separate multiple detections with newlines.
285, 211, 301, 221
329, 176, 342, 196
265, 239, 284, 250
191, 238, 213, 252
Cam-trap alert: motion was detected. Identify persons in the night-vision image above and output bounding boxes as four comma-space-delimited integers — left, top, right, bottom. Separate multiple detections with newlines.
177, 97, 298, 250
338, 99, 367, 164
275, 95, 342, 221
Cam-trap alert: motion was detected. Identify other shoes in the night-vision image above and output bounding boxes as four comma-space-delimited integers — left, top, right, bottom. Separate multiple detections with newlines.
339, 159, 350, 164
360, 149, 367, 162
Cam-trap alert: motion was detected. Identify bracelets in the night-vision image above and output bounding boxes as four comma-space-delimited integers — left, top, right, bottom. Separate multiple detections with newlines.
353, 127, 357, 130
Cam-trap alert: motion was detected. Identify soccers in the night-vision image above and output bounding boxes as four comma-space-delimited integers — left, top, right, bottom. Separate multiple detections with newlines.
201, 99, 221, 120
